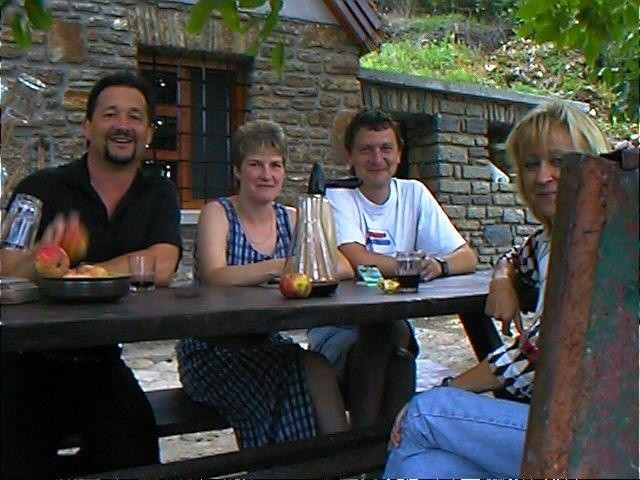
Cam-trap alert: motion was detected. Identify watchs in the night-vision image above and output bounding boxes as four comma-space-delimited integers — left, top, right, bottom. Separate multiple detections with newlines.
443, 374, 454, 387
434, 256, 448, 276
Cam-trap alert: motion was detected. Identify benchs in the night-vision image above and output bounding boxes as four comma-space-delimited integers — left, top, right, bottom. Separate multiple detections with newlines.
56, 420, 394, 479
57, 387, 231, 449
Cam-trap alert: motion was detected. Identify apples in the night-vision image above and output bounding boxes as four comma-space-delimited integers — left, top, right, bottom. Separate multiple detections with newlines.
32, 243, 69, 277
63, 262, 107, 277
278, 272, 311, 299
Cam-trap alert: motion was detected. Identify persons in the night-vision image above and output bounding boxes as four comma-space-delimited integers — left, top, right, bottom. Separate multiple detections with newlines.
307, 108, 479, 479
1, 71, 185, 477
385, 99, 610, 479
175, 120, 355, 480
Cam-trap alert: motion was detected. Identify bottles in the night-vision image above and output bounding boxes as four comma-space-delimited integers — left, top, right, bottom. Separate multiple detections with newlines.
282, 193, 340, 296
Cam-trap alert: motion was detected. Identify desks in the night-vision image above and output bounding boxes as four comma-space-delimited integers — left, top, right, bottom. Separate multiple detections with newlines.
2, 266, 526, 480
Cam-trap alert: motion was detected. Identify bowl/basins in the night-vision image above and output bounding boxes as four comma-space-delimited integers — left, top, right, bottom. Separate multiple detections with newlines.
37, 273, 131, 304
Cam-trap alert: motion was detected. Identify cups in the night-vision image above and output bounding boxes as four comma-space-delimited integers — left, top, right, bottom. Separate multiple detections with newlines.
129, 253, 158, 293
0, 193, 44, 253
397, 257, 422, 293
6, 71, 47, 126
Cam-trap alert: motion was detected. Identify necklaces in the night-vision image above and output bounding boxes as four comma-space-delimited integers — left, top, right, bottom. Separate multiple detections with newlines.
234, 195, 273, 244
